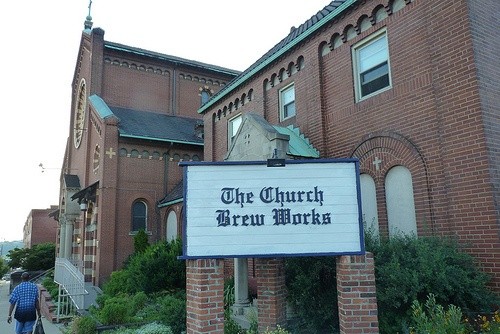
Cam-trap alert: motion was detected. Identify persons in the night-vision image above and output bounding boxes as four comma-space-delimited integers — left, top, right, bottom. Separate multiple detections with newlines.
7, 272, 42, 334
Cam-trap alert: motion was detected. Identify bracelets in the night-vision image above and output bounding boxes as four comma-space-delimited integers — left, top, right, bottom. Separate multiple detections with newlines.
8, 316, 11, 317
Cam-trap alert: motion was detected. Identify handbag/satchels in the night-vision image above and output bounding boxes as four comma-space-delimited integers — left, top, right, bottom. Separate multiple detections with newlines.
34, 317, 45, 334
